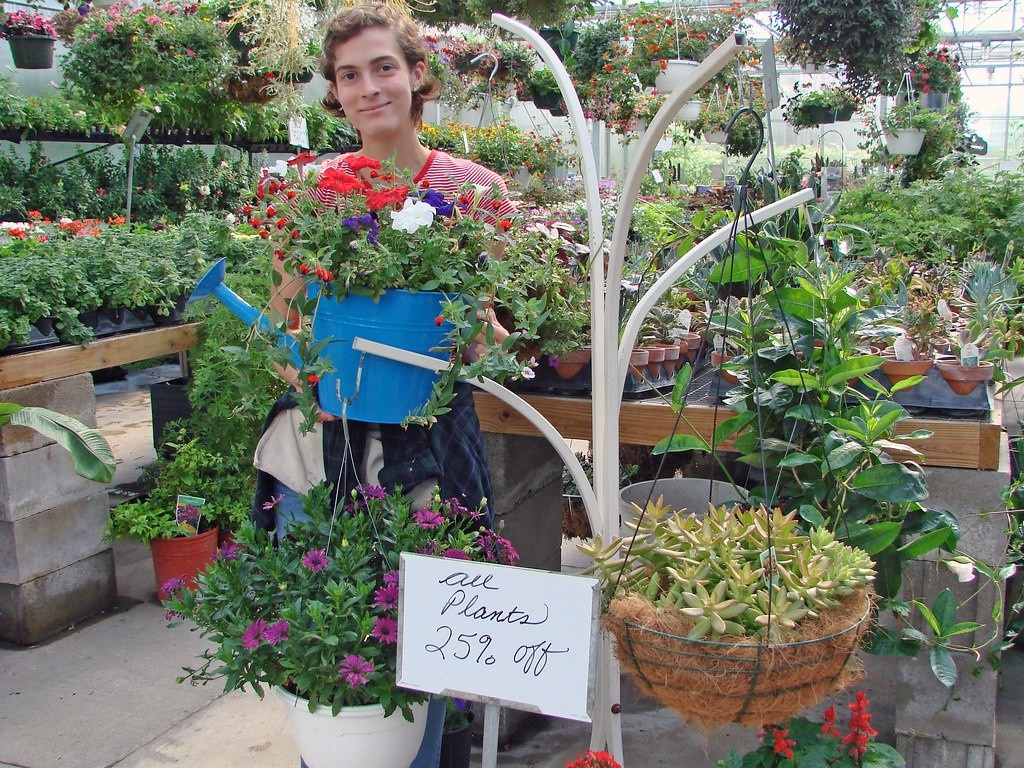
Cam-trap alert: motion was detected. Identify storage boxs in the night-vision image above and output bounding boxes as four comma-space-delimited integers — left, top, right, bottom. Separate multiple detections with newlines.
149, 372, 194, 459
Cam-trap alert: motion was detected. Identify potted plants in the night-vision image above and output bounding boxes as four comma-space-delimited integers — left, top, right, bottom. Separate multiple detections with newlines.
564, 495, 881, 731
549, 210, 995, 397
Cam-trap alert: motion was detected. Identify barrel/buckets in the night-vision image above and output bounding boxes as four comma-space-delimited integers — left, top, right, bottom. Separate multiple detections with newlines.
619, 478, 749, 537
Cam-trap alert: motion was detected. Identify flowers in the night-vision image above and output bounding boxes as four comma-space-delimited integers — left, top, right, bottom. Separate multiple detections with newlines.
157, 472, 522, 730
0, 0, 953, 387
566, 693, 909, 768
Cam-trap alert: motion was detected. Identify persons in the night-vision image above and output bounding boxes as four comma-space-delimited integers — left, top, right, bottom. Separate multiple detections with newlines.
254, 1, 518, 566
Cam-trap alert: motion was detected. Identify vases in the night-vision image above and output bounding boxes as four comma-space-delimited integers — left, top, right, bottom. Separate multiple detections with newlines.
0, 23, 927, 768
